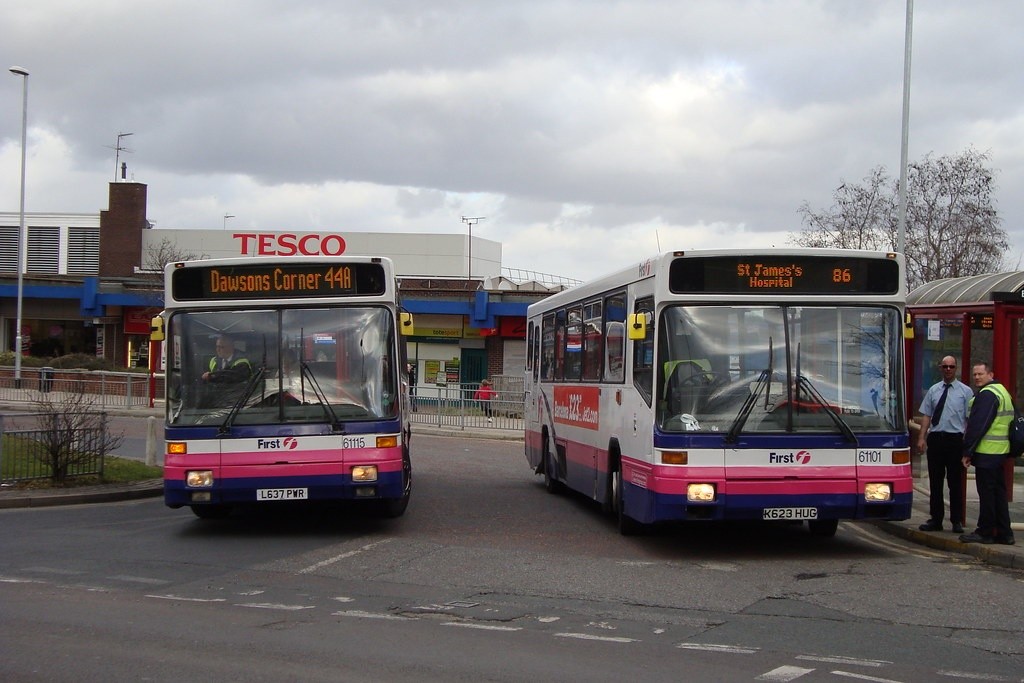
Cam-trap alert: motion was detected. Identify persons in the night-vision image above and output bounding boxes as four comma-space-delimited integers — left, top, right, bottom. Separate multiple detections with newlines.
201, 334, 254, 385
958, 360, 1024, 545
916, 355, 975, 533
274, 347, 301, 380
406, 362, 415, 406
472, 379, 498, 423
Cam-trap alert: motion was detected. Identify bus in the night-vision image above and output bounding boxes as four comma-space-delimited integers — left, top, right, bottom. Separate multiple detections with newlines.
150, 256, 413, 523
524, 247, 912, 540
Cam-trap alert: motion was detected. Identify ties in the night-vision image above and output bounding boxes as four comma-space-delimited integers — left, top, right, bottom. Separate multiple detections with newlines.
224, 360, 228, 368
932, 384, 951, 427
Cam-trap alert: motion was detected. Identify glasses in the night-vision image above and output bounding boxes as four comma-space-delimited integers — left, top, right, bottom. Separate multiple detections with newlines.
941, 364, 956, 369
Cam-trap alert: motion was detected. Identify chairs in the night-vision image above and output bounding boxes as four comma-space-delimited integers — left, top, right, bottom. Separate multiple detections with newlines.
664, 358, 713, 416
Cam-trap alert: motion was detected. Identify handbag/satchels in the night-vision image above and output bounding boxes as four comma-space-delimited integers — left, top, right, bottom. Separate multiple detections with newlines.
1006, 399, 1024, 457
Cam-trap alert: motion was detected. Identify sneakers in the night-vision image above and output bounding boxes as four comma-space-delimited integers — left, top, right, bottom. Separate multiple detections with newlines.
995, 534, 1015, 545
959, 533, 995, 544
919, 521, 943, 531
953, 522, 964, 533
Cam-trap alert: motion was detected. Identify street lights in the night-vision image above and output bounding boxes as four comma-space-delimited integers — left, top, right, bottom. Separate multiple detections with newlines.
9, 65, 29, 386
